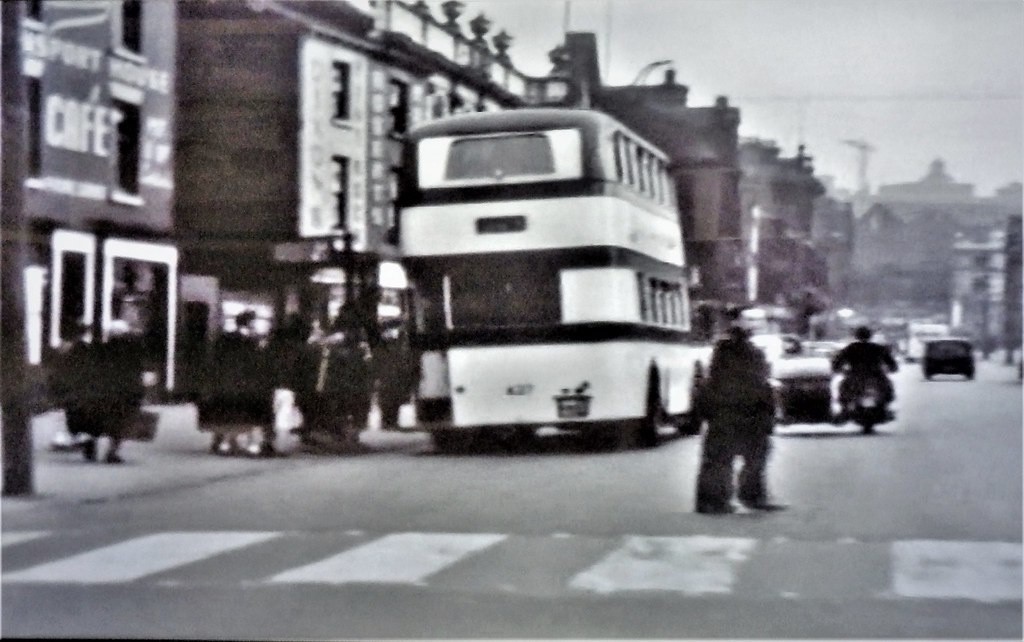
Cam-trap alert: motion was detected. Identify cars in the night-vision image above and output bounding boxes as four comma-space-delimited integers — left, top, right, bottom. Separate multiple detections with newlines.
920, 336, 976, 381
744, 311, 951, 420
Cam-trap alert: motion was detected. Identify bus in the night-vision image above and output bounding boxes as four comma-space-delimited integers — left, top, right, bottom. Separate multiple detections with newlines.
393, 111, 708, 453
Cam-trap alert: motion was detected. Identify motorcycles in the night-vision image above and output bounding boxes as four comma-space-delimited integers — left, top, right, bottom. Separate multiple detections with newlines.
829, 359, 898, 433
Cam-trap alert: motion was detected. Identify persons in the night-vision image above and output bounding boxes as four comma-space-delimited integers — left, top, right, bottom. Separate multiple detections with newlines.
832, 325, 898, 424
62, 285, 418, 460
694, 327, 784, 519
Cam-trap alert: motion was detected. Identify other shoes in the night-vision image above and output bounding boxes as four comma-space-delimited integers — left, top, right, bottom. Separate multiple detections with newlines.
85, 439, 95, 460
108, 453, 122, 463
